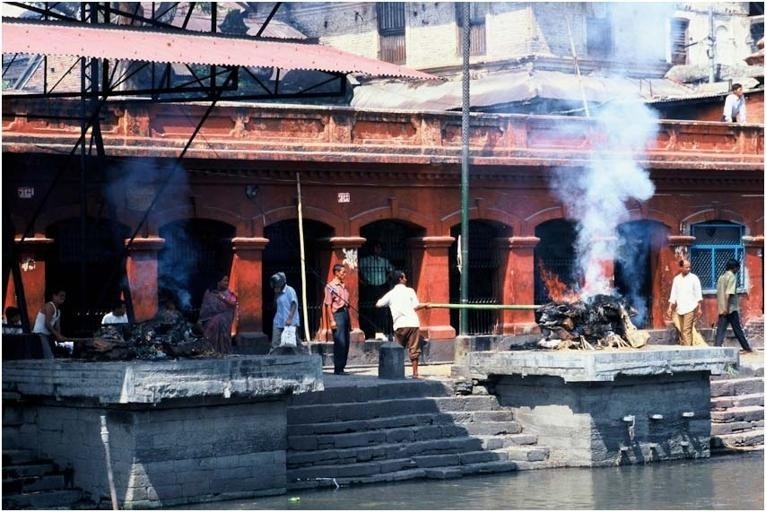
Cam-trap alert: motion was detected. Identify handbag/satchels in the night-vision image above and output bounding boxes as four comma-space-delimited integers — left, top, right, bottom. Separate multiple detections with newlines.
278, 325, 298, 348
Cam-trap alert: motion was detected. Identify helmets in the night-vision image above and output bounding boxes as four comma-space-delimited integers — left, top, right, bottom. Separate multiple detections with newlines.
269, 271, 287, 288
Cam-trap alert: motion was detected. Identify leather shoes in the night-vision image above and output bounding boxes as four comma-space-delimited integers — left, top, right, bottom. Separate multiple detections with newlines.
333, 371, 350, 375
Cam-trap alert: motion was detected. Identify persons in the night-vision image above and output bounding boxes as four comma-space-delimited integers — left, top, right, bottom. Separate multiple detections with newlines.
269, 272, 300, 355
666, 259, 703, 346
324, 264, 351, 375
375, 270, 432, 379
714, 259, 753, 353
2, 271, 240, 359
720, 84, 746, 123
